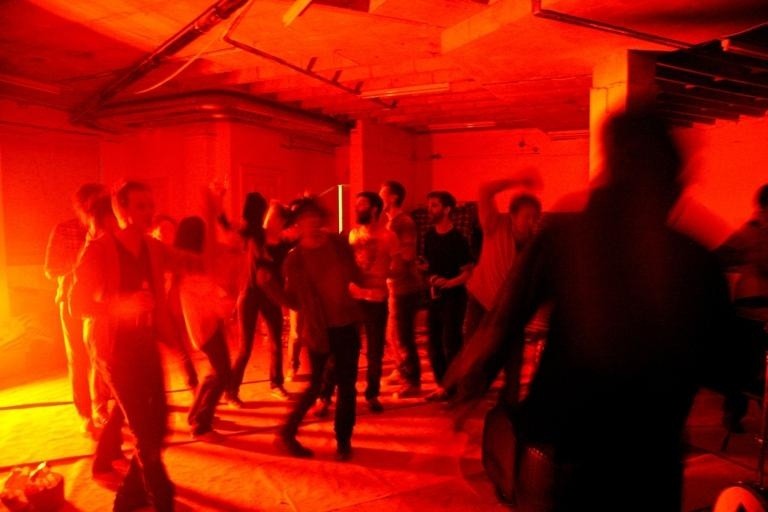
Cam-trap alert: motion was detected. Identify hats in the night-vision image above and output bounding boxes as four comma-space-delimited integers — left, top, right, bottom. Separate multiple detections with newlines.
279, 197, 309, 228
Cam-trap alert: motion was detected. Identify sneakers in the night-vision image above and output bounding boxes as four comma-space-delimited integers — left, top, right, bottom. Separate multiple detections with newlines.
225, 365, 456, 420
275, 432, 311, 457
335, 437, 352, 460
189, 430, 225, 444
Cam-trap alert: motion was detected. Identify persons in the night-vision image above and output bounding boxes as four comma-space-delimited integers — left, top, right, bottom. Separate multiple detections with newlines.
705, 183, 768, 435
423, 100, 767, 511
47, 182, 233, 512
215, 174, 544, 459
